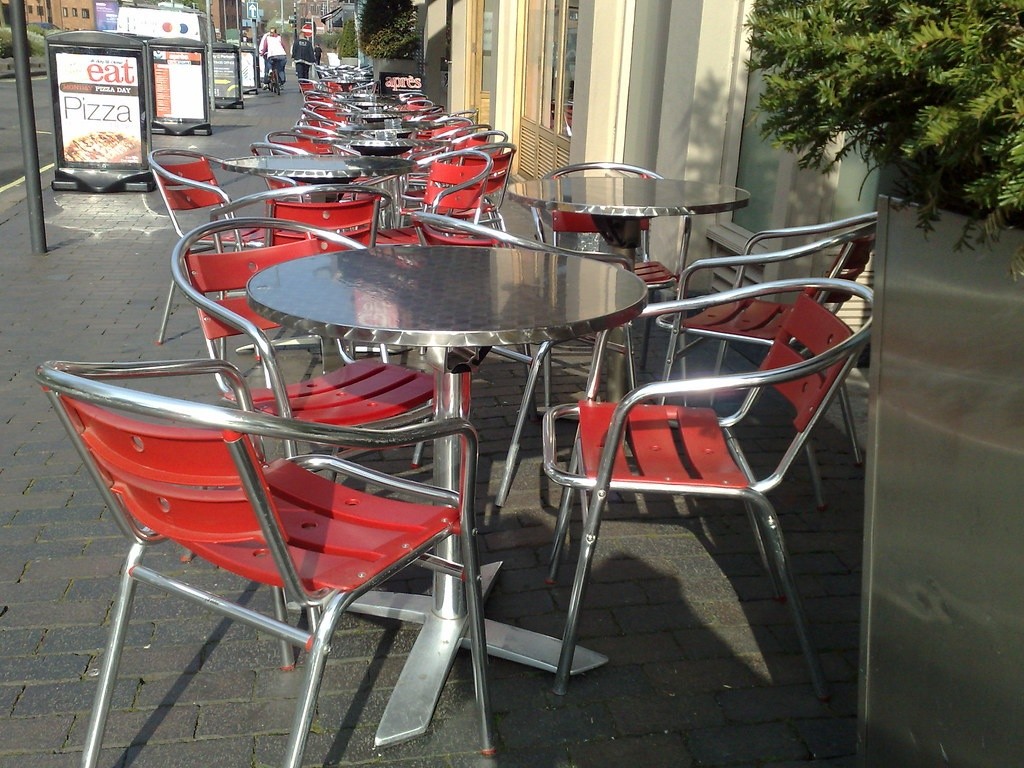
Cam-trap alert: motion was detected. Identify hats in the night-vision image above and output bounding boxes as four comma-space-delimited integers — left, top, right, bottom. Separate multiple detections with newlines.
270, 28, 276, 35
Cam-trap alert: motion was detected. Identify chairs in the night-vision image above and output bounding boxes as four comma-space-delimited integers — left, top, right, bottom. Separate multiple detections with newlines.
36, 61, 878, 768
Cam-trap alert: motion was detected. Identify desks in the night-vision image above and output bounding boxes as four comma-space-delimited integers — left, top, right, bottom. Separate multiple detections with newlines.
246, 245, 647, 745
336, 120, 445, 187
313, 133, 452, 230
337, 107, 423, 125
332, 96, 405, 110
221, 155, 420, 356
506, 177, 749, 441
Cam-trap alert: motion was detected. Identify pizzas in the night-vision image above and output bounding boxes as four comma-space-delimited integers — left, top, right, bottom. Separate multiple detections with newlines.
65, 132, 142, 163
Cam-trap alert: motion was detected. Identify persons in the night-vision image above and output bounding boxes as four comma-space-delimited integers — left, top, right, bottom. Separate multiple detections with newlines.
259, 28, 288, 90
315, 44, 322, 65
291, 32, 316, 93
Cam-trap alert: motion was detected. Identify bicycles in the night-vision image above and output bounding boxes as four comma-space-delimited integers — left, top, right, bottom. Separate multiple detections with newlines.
260, 52, 283, 95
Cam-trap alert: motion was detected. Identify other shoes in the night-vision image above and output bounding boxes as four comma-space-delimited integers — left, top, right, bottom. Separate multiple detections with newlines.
262, 84, 267, 90
